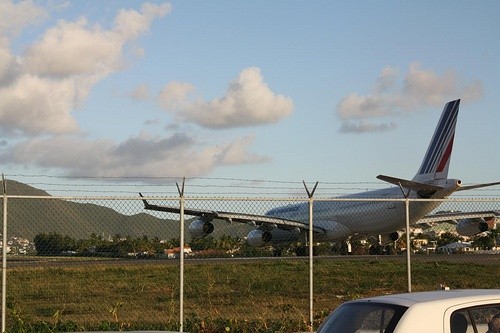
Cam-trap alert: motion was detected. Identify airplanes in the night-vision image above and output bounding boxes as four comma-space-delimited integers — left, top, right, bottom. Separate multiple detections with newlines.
139, 98, 500, 257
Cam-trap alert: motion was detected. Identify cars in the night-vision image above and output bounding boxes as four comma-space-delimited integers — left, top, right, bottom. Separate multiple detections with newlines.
315, 288, 500, 333
